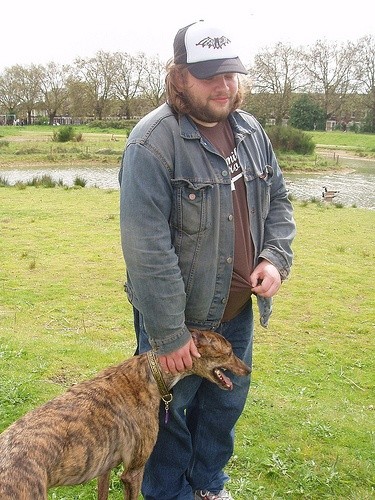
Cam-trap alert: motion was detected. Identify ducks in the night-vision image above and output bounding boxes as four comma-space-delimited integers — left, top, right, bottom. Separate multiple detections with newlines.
322, 186, 340, 198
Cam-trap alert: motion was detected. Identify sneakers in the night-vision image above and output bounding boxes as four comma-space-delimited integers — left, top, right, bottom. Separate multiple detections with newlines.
192, 487, 234, 500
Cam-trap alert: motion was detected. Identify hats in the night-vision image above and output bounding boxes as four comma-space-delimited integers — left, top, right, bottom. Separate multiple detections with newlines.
174, 20, 248, 79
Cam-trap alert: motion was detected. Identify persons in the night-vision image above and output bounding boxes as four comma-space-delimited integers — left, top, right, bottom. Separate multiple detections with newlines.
118, 17, 297, 500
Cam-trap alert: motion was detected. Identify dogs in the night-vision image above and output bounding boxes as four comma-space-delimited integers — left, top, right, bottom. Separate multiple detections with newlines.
1, 329, 252, 500
